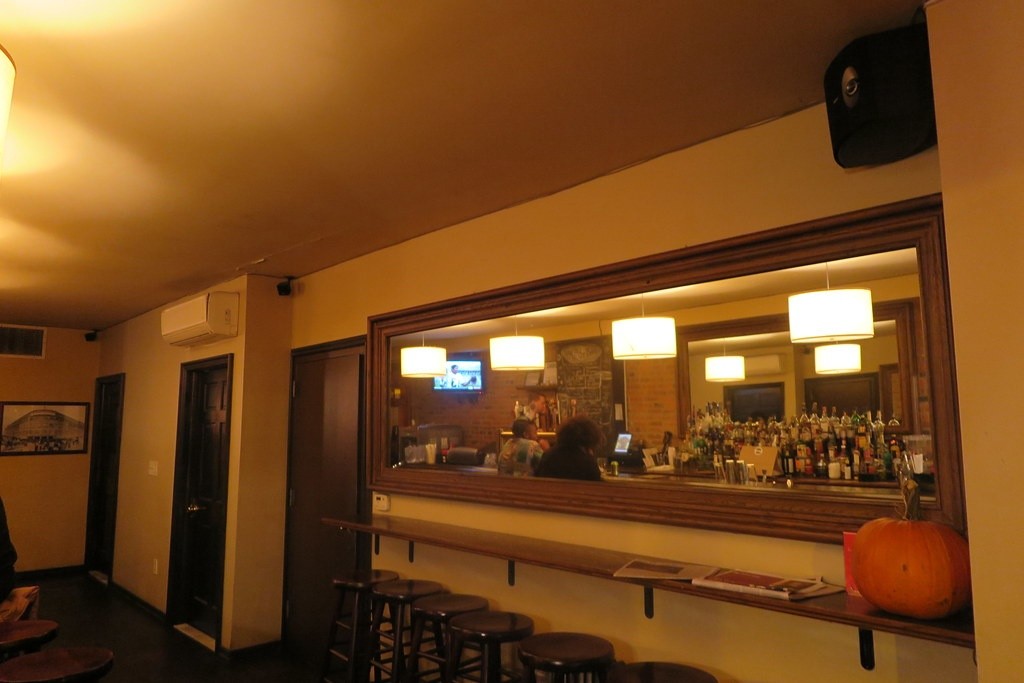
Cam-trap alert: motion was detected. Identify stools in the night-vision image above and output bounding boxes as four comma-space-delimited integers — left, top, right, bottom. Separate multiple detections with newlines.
442, 609, 536, 683
0, 620, 60, 663
612, 661, 719, 683
358, 579, 443, 683
404, 594, 489, 683
317, 569, 398, 683
517, 631, 614, 683
0, 645, 114, 683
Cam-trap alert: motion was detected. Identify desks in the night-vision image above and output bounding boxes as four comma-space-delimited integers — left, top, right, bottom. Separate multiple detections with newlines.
322, 515, 976, 670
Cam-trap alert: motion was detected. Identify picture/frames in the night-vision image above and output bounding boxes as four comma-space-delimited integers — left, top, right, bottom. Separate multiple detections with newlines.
0, 401, 90, 456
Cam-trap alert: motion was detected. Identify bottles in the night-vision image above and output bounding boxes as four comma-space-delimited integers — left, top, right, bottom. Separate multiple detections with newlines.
662, 400, 924, 485
404, 437, 447, 464
610, 461, 618, 475
514, 394, 576, 432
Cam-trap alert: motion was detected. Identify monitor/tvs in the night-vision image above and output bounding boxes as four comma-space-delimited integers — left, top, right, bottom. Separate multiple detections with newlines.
432, 358, 483, 395
614, 434, 633, 454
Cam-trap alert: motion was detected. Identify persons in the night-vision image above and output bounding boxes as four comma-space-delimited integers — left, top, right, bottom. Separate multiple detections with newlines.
441, 365, 476, 388
536, 414, 608, 480
496, 416, 550, 477
0, 498, 40, 662
530, 394, 552, 429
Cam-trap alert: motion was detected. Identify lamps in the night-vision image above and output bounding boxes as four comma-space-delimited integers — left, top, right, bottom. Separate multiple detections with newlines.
814, 342, 861, 375
489, 318, 545, 371
400, 332, 447, 378
705, 340, 746, 382
787, 263, 875, 344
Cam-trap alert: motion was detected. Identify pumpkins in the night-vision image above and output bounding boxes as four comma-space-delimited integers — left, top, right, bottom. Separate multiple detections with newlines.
852, 480, 970, 618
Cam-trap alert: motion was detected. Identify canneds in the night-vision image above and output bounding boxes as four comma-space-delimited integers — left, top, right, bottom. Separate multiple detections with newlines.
610, 461, 619, 476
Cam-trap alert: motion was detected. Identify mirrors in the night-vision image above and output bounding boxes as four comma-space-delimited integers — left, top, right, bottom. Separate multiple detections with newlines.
675, 297, 914, 437
367, 191, 966, 552
612, 297, 679, 362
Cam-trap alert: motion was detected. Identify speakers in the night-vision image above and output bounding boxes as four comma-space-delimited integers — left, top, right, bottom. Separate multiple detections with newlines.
85, 332, 97, 342
277, 280, 291, 296
823, 22, 938, 170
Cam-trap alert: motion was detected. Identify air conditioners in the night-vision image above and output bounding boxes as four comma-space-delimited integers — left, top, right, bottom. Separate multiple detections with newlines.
161, 291, 238, 347
